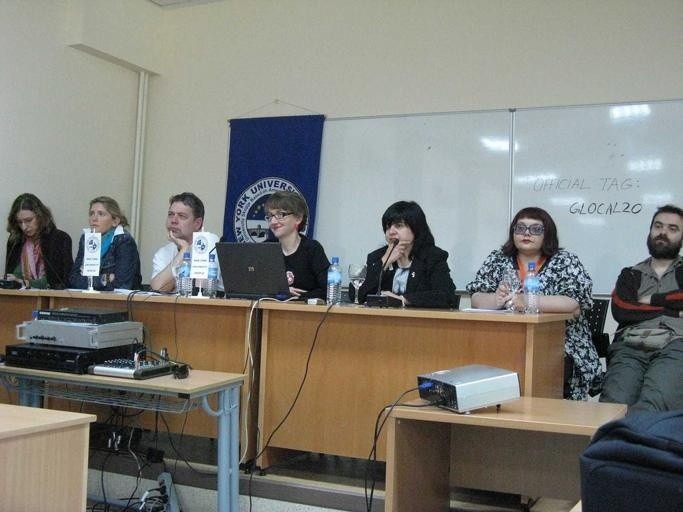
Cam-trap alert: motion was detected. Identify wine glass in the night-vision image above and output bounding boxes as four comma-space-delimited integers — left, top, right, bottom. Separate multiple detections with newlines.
502, 269, 520, 313
349, 264, 368, 305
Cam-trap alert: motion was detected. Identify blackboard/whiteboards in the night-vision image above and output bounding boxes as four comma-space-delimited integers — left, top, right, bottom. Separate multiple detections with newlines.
314, 99, 681, 297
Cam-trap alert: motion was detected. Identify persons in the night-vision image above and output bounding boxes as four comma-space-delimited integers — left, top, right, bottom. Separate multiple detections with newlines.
259, 190, 331, 302
68, 195, 145, 291
0, 193, 74, 289
149, 192, 226, 294
465, 207, 604, 403
598, 205, 682, 413
348, 200, 458, 309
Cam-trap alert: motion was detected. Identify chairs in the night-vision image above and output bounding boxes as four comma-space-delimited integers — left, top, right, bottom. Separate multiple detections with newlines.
585, 298, 610, 374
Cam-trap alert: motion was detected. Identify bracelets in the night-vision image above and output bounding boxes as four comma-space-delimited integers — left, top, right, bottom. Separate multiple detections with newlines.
104, 272, 110, 286
399, 295, 405, 308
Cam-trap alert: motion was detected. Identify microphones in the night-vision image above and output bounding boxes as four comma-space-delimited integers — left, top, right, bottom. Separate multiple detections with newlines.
191, 235, 227, 298
367, 239, 402, 308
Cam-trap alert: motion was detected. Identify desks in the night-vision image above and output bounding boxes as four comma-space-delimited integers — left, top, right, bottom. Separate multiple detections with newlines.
384, 384, 629, 512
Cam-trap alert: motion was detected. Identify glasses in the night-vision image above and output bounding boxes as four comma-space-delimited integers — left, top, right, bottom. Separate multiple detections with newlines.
265, 212, 295, 220
513, 224, 544, 235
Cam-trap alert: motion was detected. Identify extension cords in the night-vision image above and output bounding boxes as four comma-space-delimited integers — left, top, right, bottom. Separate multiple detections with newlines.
158, 472, 180, 512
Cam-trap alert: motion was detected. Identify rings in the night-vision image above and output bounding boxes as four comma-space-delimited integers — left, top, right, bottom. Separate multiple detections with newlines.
510, 306, 514, 311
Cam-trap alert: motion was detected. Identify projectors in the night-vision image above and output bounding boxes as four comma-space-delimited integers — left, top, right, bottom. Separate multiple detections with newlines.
416, 363, 522, 413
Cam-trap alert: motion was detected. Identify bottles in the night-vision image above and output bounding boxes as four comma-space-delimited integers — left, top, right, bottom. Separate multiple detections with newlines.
172, 252, 218, 300
327, 257, 342, 304
523, 261, 541, 315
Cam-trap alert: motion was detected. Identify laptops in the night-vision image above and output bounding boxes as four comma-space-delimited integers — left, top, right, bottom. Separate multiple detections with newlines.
214, 242, 307, 301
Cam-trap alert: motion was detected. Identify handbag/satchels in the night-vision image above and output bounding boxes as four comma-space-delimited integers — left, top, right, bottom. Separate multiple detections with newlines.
623, 328, 671, 348
